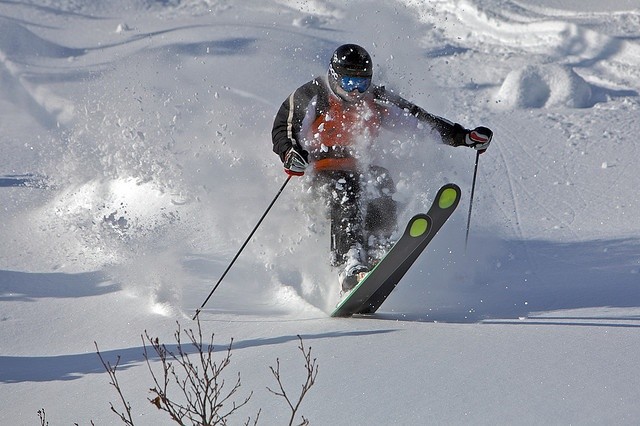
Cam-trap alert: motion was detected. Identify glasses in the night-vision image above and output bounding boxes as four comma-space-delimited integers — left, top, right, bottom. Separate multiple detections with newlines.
328, 65, 371, 92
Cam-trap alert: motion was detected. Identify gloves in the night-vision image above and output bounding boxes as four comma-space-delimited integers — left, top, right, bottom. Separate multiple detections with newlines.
283, 144, 310, 176
465, 126, 494, 153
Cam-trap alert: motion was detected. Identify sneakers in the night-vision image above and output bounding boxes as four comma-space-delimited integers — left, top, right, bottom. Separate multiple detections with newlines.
364, 238, 396, 270
336, 263, 369, 293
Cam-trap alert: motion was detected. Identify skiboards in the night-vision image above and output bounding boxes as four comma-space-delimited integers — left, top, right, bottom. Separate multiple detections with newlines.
330, 182, 461, 317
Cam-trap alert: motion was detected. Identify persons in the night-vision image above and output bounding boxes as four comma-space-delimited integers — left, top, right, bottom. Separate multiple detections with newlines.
271, 44, 493, 299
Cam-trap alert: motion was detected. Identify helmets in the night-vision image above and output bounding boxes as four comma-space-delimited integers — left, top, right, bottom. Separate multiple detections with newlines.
326, 43, 373, 105
